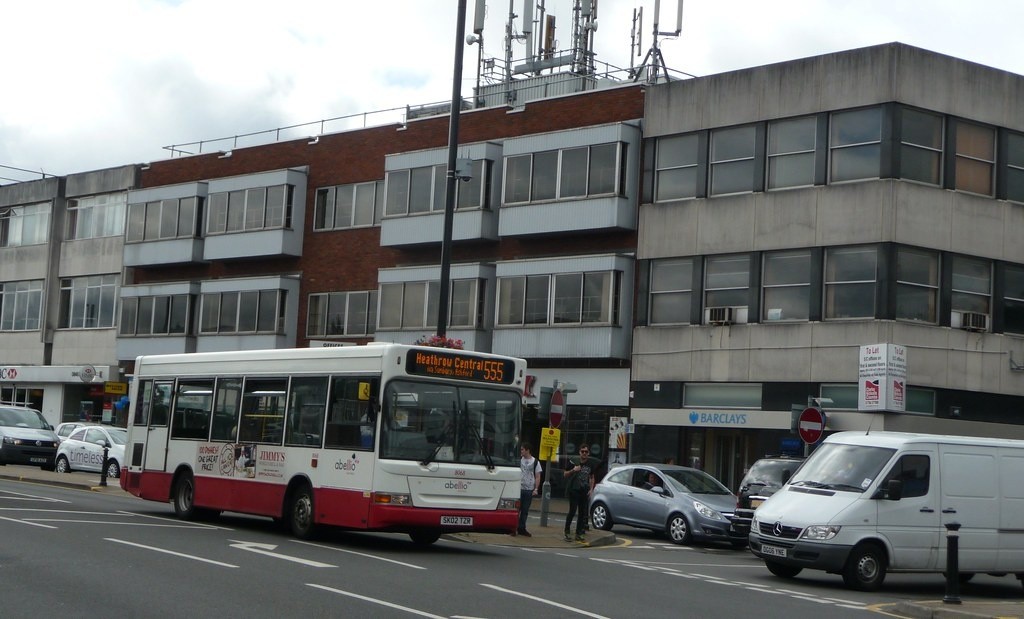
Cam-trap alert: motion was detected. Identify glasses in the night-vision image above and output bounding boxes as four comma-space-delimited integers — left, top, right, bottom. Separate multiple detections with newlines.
580, 450, 589, 454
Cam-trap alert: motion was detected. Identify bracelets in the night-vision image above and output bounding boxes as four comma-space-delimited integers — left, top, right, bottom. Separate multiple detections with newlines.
535, 489, 538, 490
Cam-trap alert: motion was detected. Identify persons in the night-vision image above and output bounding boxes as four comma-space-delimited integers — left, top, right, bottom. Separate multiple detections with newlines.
361, 412, 375, 448
644, 471, 662, 491
564, 443, 595, 545
519, 442, 543, 538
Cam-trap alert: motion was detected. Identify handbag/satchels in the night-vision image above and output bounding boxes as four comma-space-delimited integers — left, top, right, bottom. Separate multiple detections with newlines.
566, 473, 580, 498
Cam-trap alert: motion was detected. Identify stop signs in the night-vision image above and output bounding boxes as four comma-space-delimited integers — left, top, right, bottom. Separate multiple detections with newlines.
548, 389, 564, 427
797, 405, 823, 445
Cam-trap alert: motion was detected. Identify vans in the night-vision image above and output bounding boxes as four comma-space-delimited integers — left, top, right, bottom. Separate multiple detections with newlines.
748, 418, 1024, 592
0, 403, 61, 472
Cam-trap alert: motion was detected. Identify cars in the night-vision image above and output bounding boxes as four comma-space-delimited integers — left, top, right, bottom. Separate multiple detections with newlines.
539, 455, 608, 495
587, 462, 748, 547
54, 426, 127, 478
55, 422, 112, 443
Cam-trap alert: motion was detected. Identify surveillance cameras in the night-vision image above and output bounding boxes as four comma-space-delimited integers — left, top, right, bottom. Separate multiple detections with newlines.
455, 158, 474, 182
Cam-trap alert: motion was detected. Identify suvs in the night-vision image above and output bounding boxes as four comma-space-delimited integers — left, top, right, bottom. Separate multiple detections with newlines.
729, 454, 808, 535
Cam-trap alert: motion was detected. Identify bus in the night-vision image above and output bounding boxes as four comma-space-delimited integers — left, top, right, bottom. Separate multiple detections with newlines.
117, 342, 538, 545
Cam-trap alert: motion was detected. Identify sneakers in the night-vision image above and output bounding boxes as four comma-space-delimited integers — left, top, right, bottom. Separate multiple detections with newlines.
518, 528, 531, 537
575, 534, 589, 542
563, 531, 572, 542
